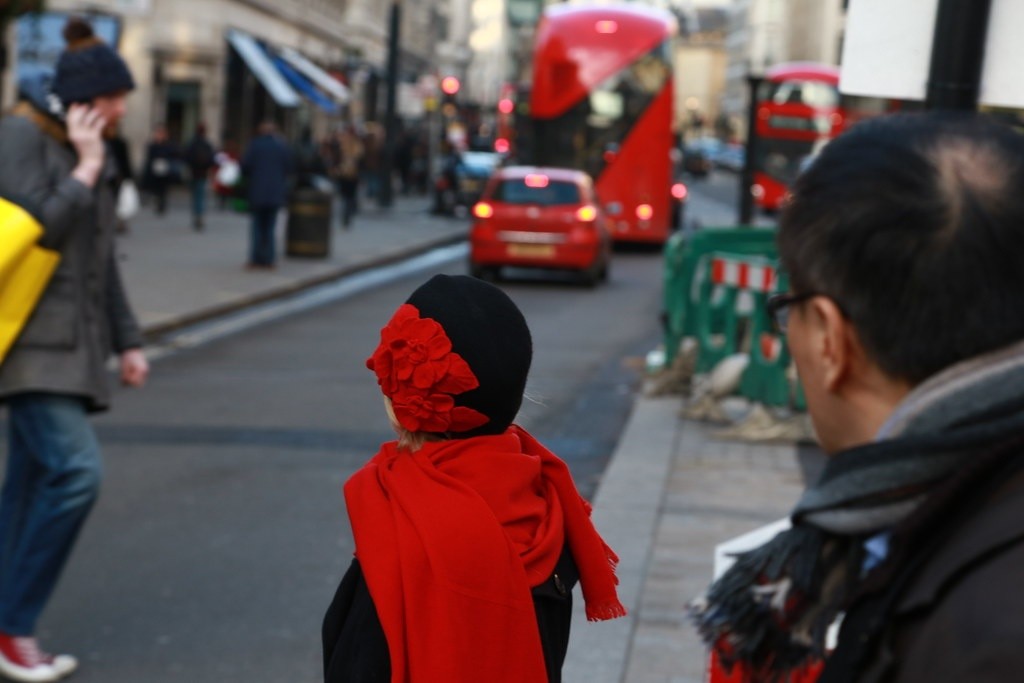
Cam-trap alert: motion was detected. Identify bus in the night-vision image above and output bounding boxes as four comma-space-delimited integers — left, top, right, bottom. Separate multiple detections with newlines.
751, 65, 898, 215
443, 94, 516, 198
526, 0, 678, 250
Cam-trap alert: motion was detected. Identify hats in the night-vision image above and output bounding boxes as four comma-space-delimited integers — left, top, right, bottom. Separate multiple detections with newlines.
365, 273, 532, 438
45, 17, 135, 113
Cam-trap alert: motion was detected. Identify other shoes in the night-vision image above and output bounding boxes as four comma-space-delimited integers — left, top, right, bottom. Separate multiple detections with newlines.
0, 634, 78, 683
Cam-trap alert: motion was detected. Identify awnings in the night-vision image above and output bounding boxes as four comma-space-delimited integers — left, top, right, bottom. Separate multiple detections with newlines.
230, 32, 350, 110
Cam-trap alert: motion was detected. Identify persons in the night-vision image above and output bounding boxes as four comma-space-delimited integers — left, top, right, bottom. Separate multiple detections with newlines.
103, 80, 816, 268
0, 17, 152, 683
681, 108, 1023, 683
321, 273, 579, 683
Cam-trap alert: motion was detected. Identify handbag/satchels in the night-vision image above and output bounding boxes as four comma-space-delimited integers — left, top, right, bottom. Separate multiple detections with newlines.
2, 195, 64, 367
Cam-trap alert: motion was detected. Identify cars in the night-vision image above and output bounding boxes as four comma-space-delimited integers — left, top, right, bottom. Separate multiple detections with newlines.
466, 165, 621, 286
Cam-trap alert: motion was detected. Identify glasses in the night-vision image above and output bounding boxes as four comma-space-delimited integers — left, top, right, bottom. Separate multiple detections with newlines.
766, 287, 848, 336
